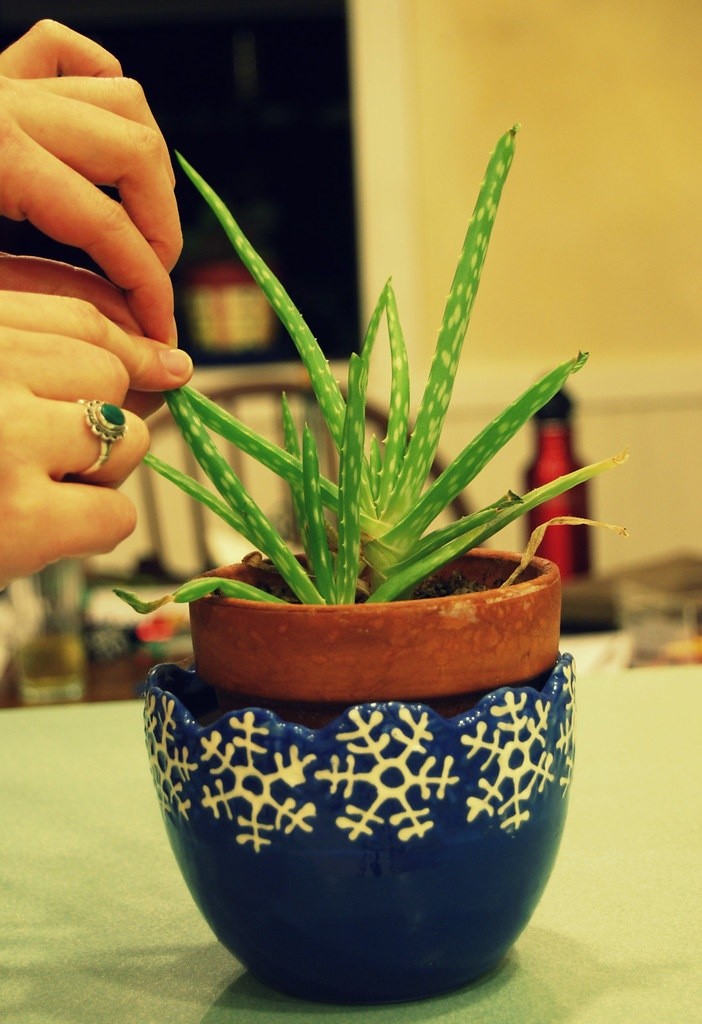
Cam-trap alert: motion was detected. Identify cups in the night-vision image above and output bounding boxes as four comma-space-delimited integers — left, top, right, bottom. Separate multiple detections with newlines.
12, 558, 88, 705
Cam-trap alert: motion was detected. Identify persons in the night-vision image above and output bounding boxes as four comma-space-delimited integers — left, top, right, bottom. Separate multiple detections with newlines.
0, 19, 194, 598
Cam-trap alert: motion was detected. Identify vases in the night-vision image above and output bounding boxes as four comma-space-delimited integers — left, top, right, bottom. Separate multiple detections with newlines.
141, 648, 577, 1006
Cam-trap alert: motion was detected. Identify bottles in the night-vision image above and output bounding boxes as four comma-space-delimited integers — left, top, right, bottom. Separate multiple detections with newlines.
523, 391, 591, 579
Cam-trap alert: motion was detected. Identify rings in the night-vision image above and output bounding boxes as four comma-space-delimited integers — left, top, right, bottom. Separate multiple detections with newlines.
73, 398, 126, 478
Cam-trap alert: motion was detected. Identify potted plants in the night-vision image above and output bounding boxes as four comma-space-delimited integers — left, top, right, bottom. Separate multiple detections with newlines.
112, 121, 633, 728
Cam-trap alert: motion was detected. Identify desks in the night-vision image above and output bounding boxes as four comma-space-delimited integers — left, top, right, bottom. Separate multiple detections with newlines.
0, 632, 702, 1024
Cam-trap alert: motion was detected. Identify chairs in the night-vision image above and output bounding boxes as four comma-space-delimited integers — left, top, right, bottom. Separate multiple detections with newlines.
141, 382, 484, 577
